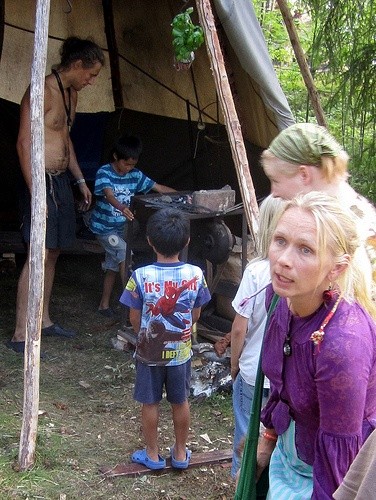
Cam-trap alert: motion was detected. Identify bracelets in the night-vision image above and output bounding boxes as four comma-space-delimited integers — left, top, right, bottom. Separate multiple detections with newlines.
73, 179, 86, 187
259, 431, 279, 444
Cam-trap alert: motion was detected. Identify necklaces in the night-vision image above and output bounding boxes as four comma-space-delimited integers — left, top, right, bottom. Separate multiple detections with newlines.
52, 69, 74, 133
281, 298, 328, 358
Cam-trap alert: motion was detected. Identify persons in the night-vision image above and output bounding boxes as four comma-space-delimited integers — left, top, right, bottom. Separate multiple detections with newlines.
228, 189, 286, 483
118, 207, 212, 470
232, 190, 376, 500
87, 141, 178, 318
4, 35, 106, 354
261, 122, 376, 326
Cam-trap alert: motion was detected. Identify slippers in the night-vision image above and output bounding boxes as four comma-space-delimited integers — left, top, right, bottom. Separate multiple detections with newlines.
171, 448, 191, 469
129, 448, 168, 469
41, 321, 78, 338
100, 308, 114, 318
7, 341, 25, 352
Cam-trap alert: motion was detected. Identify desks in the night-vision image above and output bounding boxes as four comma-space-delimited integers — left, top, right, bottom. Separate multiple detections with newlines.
121, 190, 248, 340
